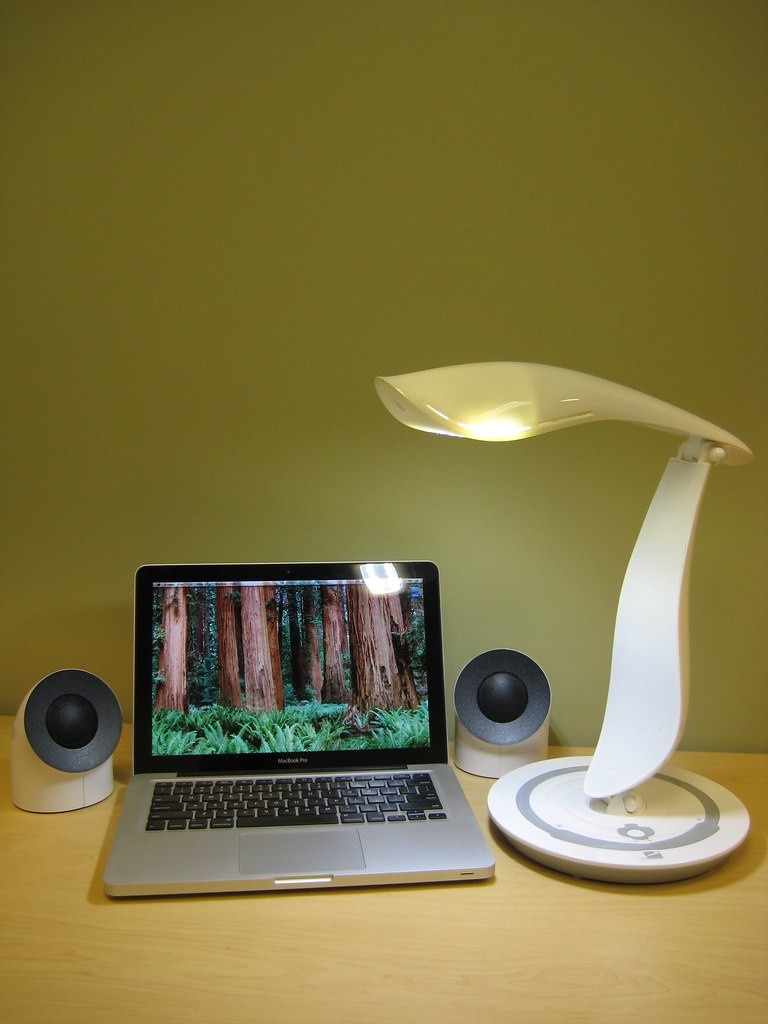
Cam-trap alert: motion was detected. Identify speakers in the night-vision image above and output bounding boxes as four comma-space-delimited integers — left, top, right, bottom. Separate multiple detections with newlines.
453, 648, 552, 778
11, 668, 123, 814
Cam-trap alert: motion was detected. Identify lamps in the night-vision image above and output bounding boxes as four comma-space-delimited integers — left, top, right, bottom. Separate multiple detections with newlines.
375, 361, 755, 884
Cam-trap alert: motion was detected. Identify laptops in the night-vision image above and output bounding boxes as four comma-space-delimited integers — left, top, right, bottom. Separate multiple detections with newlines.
103, 560, 495, 898
